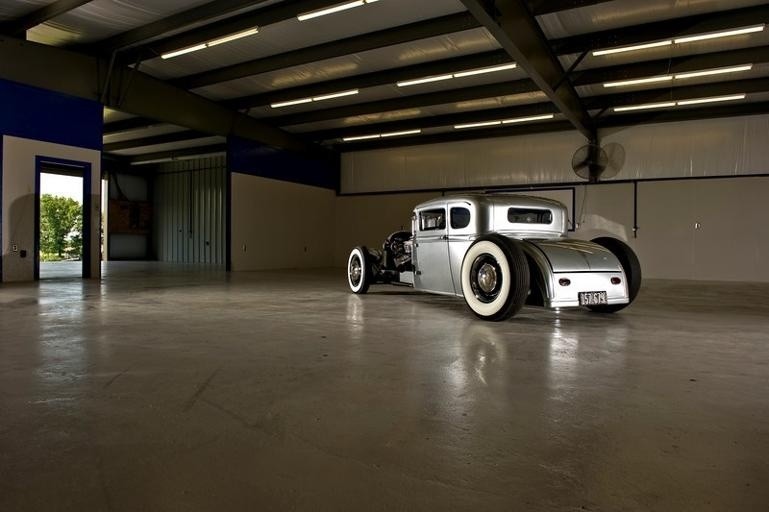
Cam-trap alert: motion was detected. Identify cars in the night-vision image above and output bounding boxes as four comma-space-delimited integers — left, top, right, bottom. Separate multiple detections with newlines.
348, 194, 641, 321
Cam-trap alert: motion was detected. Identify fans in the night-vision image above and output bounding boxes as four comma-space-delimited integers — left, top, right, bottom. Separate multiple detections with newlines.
569, 144, 608, 179
